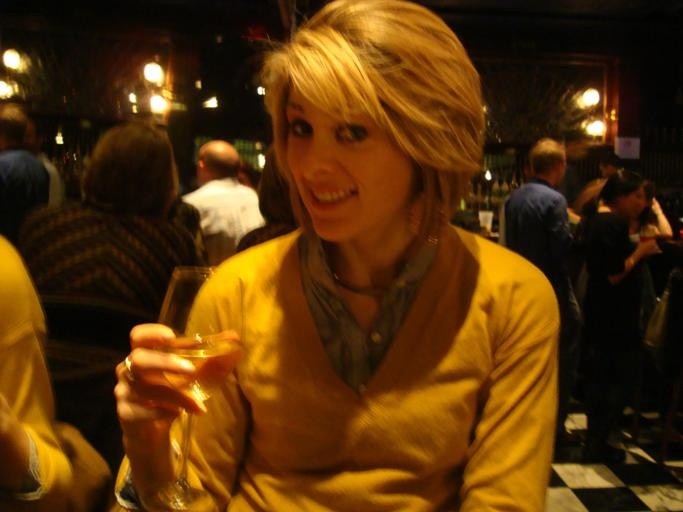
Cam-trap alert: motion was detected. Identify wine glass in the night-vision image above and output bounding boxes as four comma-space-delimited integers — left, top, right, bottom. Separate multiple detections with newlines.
149, 265, 246, 512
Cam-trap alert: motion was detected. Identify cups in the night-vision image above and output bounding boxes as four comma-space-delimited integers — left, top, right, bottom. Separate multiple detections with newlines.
639, 227, 656, 242
476, 209, 494, 237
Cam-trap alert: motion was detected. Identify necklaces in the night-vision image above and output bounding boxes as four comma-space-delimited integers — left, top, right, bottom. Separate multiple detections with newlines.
329, 267, 385, 297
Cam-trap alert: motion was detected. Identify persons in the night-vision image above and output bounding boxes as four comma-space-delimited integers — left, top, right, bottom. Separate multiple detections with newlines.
449, 138, 680, 464
115, 1, 564, 512
2, 105, 302, 509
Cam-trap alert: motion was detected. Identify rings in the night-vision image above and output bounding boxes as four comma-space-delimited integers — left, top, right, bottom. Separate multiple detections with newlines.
119, 355, 136, 378
125, 356, 135, 381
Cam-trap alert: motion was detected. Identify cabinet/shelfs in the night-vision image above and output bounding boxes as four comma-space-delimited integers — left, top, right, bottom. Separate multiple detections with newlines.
473, 55, 643, 158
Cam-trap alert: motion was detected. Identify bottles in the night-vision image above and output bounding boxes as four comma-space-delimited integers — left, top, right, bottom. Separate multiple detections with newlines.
642, 270, 673, 352
462, 179, 521, 213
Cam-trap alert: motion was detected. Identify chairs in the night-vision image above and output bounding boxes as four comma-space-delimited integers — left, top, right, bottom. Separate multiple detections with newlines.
39, 291, 157, 478
629, 270, 683, 472
